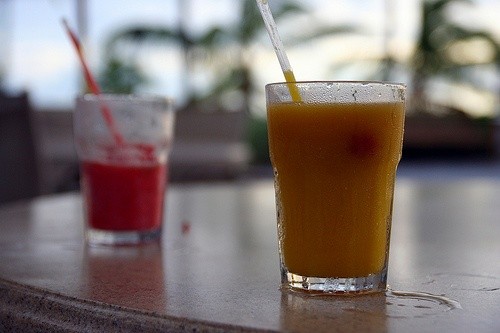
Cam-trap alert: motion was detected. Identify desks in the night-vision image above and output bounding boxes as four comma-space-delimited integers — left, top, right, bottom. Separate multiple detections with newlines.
0, 177, 500, 333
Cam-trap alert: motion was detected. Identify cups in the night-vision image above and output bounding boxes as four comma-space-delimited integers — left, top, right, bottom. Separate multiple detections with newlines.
265, 81, 408, 294
74, 94, 175, 244
